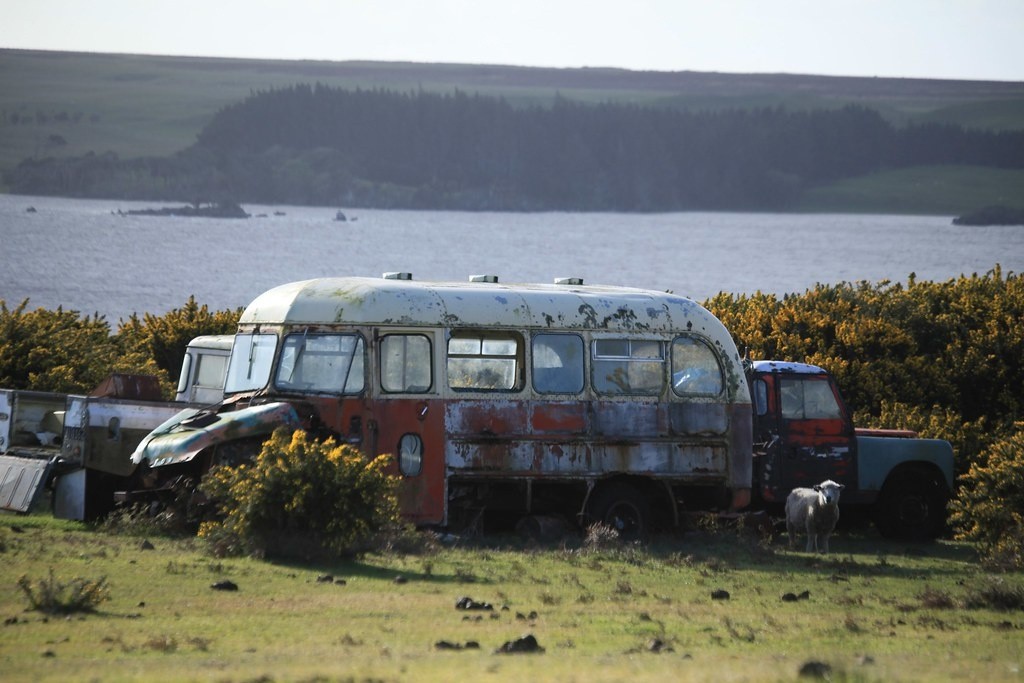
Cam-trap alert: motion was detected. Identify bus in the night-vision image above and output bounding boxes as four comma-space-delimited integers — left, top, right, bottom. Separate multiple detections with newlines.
2, 275, 959, 542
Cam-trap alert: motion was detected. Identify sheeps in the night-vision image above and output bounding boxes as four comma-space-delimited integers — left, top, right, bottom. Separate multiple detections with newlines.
784, 479, 846, 555
